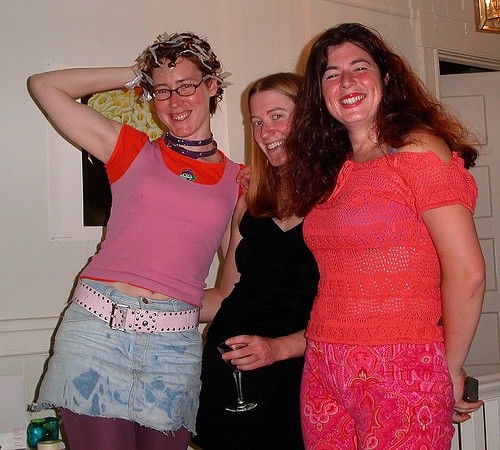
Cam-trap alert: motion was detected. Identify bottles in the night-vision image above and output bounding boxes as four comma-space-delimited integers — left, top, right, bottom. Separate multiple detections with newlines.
27, 417, 59, 448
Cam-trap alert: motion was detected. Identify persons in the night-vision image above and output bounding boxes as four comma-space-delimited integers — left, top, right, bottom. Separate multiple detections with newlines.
281, 21, 486, 450
28, 32, 250, 450
192, 71, 321, 450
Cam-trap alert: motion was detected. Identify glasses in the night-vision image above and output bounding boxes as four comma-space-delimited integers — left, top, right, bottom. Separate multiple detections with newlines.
149, 75, 214, 101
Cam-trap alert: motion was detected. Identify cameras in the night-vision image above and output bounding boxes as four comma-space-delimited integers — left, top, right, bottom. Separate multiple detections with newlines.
462, 376, 479, 403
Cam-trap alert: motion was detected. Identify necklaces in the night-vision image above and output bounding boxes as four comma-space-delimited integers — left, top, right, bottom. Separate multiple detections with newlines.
165, 131, 218, 158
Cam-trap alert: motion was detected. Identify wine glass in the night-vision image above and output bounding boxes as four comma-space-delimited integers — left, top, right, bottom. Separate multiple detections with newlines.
217, 342, 258, 413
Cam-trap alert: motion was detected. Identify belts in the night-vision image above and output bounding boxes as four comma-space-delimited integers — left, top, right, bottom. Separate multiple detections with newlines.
70, 279, 200, 334
